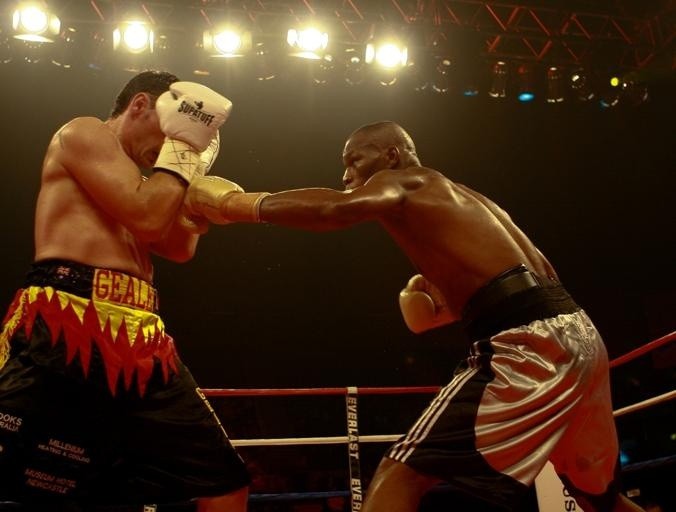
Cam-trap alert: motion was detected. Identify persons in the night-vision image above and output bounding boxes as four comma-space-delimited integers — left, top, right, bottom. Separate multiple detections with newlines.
188, 118, 646, 511
2, 69, 262, 512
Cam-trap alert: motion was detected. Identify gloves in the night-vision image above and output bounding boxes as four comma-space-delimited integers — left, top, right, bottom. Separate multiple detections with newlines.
398, 274, 462, 334
154, 81, 267, 224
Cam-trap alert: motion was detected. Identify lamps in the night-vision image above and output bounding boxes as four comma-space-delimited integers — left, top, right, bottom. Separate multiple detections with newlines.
9, 0, 650, 110
